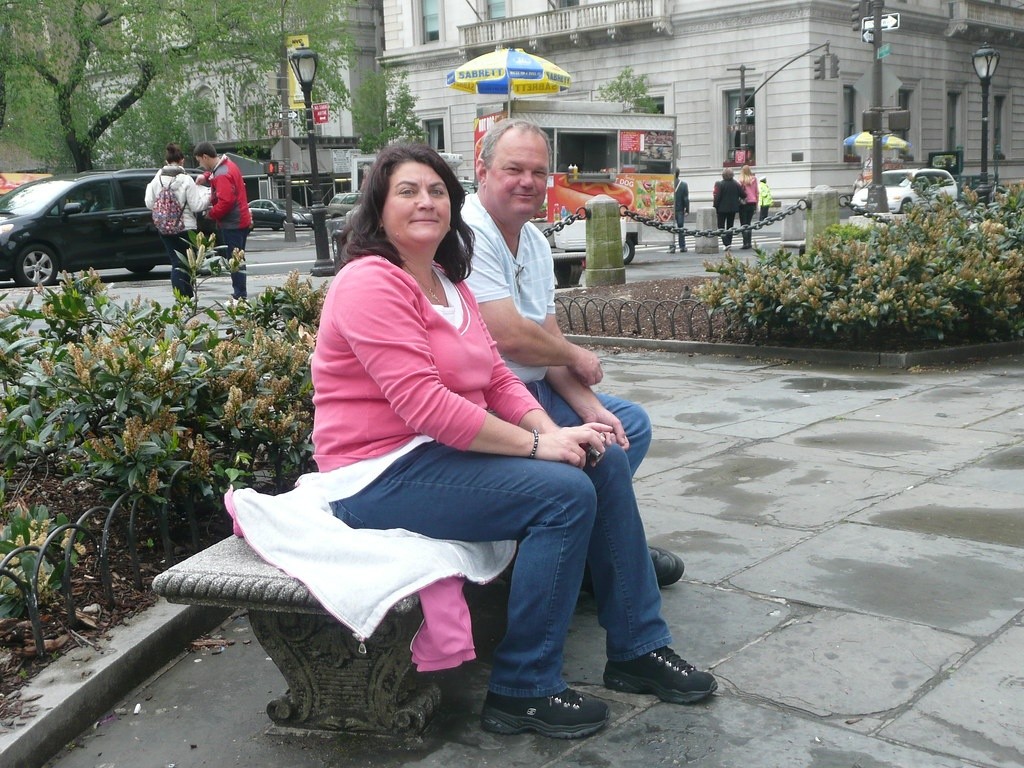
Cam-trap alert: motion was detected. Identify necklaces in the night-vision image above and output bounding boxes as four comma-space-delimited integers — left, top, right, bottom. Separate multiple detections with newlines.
402, 259, 442, 305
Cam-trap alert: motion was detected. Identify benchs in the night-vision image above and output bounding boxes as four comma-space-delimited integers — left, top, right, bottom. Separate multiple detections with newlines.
151, 532, 500, 744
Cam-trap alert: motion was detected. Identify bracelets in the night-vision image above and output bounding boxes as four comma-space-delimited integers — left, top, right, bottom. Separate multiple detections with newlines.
529, 429, 538, 459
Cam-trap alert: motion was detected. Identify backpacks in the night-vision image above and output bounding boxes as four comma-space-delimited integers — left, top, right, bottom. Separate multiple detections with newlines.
152, 175, 185, 235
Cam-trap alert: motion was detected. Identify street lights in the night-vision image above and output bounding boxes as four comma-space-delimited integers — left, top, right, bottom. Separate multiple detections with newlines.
972, 39, 1002, 207
288, 37, 337, 279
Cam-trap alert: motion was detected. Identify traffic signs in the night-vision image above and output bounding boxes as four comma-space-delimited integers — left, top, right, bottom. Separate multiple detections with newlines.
861, 13, 900, 33
729, 106, 755, 131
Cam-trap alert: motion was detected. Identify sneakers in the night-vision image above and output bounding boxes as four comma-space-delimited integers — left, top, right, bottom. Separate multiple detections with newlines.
482, 687, 610, 738
603, 646, 718, 702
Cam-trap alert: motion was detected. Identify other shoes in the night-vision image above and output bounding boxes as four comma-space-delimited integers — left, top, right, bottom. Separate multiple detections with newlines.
741, 243, 749, 249
748, 243, 752, 247
723, 244, 731, 251
680, 248, 687, 252
648, 545, 685, 587
231, 294, 248, 301
668, 246, 676, 253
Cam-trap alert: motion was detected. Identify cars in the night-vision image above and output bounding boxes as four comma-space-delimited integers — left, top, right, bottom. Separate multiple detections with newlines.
327, 191, 365, 219
246, 199, 317, 230
850, 167, 957, 216
0, 167, 233, 286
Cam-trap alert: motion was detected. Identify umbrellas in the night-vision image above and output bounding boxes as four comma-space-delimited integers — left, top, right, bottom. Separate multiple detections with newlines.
446, 48, 570, 118
844, 131, 912, 151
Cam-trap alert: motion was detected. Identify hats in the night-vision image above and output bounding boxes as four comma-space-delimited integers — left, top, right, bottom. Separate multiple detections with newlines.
760, 175, 767, 181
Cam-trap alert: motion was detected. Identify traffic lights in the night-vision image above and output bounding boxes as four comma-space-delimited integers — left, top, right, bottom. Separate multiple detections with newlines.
851, 0, 868, 35
265, 161, 280, 175
813, 56, 825, 80
830, 54, 840, 80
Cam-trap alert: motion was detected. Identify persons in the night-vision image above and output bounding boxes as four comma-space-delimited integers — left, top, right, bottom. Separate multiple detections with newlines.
193, 142, 253, 307
311, 145, 613, 740
144, 143, 212, 306
667, 167, 689, 252
454, 118, 685, 598
713, 165, 774, 250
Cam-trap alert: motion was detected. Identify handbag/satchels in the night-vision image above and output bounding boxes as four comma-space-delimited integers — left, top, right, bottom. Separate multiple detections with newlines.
738, 196, 748, 205
